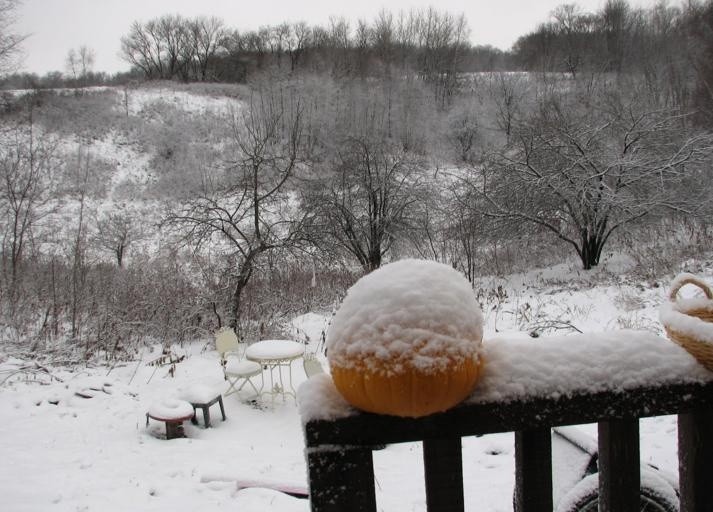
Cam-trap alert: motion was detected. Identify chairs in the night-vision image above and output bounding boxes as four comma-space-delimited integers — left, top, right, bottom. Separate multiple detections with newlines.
215, 327, 327, 406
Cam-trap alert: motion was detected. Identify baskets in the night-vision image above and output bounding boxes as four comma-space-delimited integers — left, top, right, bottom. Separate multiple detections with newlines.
663, 278, 712, 373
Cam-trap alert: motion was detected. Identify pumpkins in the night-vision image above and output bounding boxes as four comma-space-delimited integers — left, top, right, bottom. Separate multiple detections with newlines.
329, 325, 484, 418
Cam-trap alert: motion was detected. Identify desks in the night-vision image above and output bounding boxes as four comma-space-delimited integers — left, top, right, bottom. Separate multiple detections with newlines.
146, 395, 226, 440
